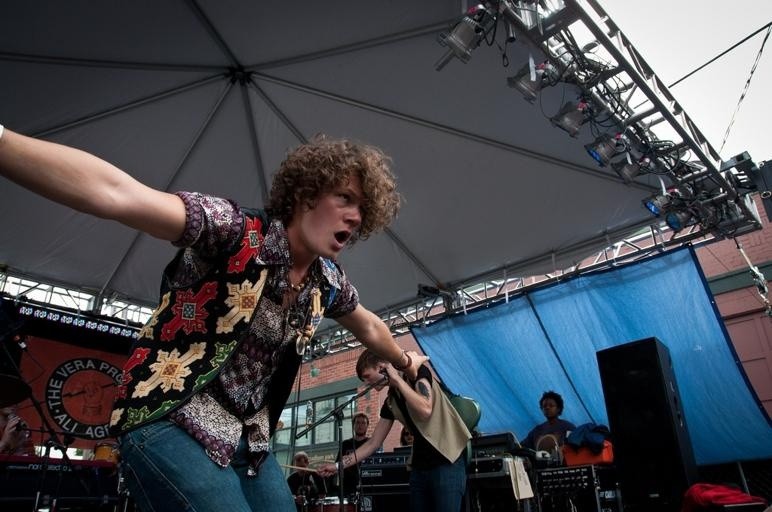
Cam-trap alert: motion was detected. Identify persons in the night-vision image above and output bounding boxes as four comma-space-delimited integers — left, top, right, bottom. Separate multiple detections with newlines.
283, 450, 334, 497
0, 121, 434, 511
397, 426, 415, 446
313, 347, 468, 511
2, 373, 36, 458
336, 412, 377, 495
515, 390, 579, 455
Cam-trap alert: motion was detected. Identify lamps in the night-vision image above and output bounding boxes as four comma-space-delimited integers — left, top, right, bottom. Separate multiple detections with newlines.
549, 97, 596, 139
434, 2, 502, 72
609, 153, 642, 185
642, 188, 681, 218
666, 206, 693, 232
507, 60, 554, 106
583, 131, 627, 168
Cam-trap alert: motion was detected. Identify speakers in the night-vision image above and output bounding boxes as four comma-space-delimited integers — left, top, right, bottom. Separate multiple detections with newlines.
592, 337, 699, 511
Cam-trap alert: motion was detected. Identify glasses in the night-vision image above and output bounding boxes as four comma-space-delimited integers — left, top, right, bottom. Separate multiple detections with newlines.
542, 402, 557, 408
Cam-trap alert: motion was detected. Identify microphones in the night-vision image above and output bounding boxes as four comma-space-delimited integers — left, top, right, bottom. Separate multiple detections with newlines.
0, 308, 27, 352
6, 414, 27, 433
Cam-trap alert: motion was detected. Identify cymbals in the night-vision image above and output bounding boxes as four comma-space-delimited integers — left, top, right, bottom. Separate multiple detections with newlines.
0, 374, 32, 409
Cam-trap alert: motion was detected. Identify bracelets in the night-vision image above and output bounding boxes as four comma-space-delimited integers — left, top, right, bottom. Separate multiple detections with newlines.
392, 352, 413, 371
0, 124, 6, 139
335, 461, 339, 469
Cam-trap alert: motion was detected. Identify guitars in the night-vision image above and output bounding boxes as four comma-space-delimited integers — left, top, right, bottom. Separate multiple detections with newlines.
446, 394, 481, 470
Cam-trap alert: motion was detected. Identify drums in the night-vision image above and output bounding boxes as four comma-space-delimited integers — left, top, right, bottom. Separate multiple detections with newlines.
292, 495, 315, 512
94, 440, 120, 468
311, 495, 359, 511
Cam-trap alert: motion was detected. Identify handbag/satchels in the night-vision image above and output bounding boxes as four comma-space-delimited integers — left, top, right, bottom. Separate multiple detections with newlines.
448, 393, 481, 433
